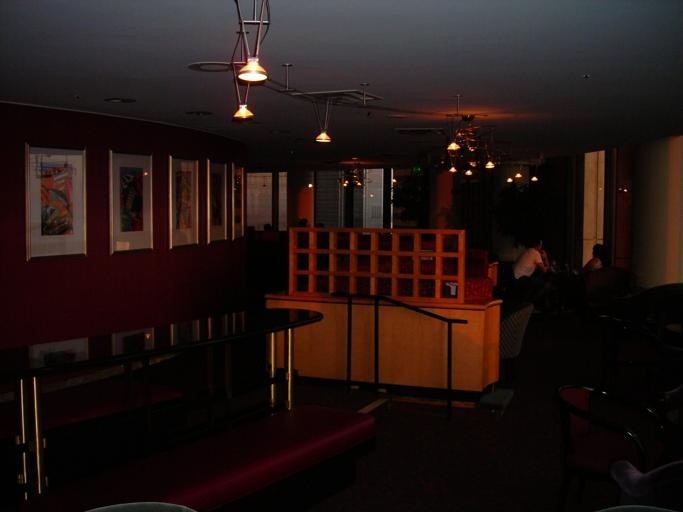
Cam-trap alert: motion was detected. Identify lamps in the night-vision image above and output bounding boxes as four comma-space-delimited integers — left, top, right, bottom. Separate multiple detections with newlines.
311, 99, 332, 142
232, 61, 255, 119
445, 114, 540, 183
343, 154, 366, 189
237, 0, 269, 82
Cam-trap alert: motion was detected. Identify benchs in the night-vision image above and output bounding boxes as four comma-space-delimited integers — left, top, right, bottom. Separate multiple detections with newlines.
29, 401, 375, 512
0, 374, 182, 489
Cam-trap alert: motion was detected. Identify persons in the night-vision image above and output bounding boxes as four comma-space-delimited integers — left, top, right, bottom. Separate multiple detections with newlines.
510, 236, 554, 281
581, 240, 606, 268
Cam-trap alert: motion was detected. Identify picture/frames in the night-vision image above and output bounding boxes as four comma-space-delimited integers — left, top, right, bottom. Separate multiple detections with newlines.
109, 150, 154, 256
206, 159, 227, 243
231, 163, 245, 241
169, 156, 199, 250
26, 141, 87, 260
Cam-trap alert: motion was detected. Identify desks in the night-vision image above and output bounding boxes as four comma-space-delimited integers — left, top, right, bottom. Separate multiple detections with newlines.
0, 308, 324, 511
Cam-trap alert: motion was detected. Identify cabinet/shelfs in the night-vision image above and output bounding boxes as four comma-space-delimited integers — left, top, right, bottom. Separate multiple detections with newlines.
288, 226, 468, 306
